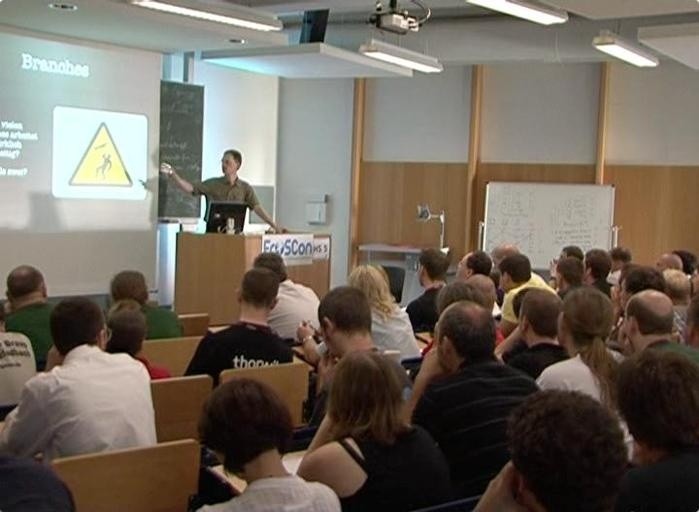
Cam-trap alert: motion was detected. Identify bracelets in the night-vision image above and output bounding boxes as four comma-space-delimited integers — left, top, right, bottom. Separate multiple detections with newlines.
301, 335, 312, 344
166, 164, 173, 177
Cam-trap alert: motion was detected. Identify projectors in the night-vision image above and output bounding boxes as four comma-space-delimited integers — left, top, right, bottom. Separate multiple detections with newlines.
365, 11, 418, 35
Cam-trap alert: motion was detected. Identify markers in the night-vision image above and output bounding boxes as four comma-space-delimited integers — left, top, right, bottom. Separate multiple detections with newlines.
158, 219, 178, 224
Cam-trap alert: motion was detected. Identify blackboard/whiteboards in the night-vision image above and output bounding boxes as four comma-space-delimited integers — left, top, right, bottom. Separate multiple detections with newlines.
482, 180, 616, 272
158, 79, 205, 224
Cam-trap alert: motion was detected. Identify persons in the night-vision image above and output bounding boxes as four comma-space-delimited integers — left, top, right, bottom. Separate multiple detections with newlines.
254, 251, 322, 349
185, 266, 293, 388
408, 240, 698, 511
2, 264, 187, 406
302, 286, 379, 431
196, 376, 343, 511
160, 149, 290, 234
295, 263, 422, 367
0, 296, 158, 464
2, 450, 77, 511
293, 346, 452, 512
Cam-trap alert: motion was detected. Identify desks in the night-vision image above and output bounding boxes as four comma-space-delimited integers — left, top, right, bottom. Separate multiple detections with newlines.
358, 243, 436, 306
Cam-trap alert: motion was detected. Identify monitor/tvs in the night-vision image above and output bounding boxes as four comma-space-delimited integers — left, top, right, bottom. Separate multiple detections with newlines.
206, 200, 247, 234
